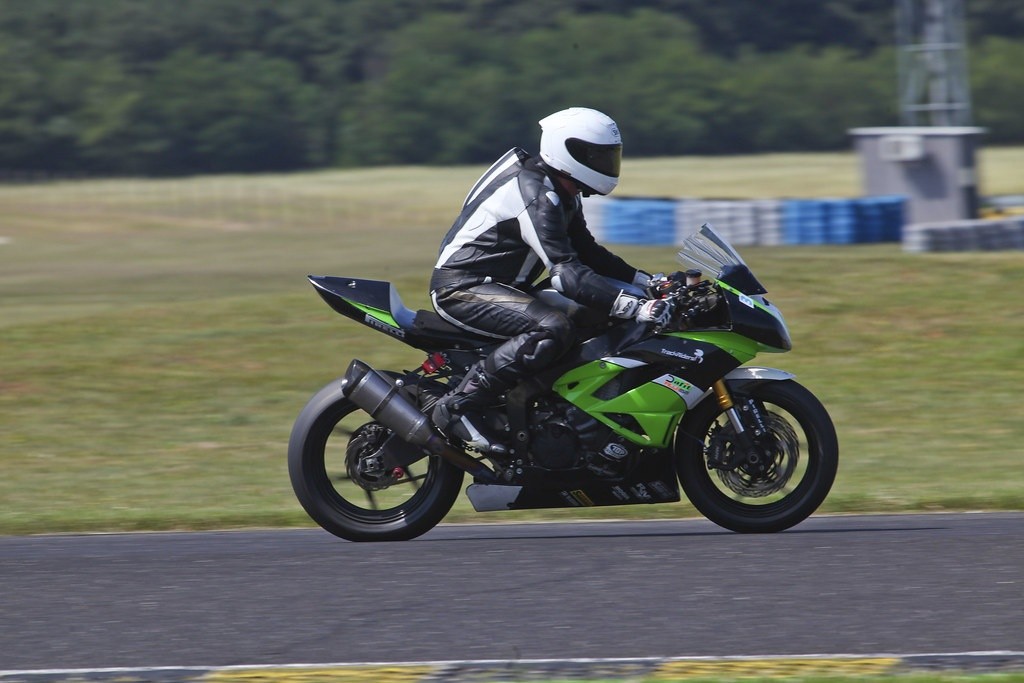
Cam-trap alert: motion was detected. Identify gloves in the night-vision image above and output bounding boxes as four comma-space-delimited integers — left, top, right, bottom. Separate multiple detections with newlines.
632, 268, 665, 290
609, 287, 673, 333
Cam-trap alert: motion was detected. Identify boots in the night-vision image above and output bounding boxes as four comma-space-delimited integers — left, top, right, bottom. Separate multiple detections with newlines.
431, 360, 493, 451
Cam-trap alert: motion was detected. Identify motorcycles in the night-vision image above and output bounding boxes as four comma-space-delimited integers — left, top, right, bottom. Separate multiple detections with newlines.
283, 218, 842, 545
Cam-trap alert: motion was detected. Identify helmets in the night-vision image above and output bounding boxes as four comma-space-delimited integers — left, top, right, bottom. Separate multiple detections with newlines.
539, 108, 622, 196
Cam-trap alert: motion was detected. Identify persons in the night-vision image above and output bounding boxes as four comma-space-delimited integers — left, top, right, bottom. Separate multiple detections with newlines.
431, 107, 698, 454
